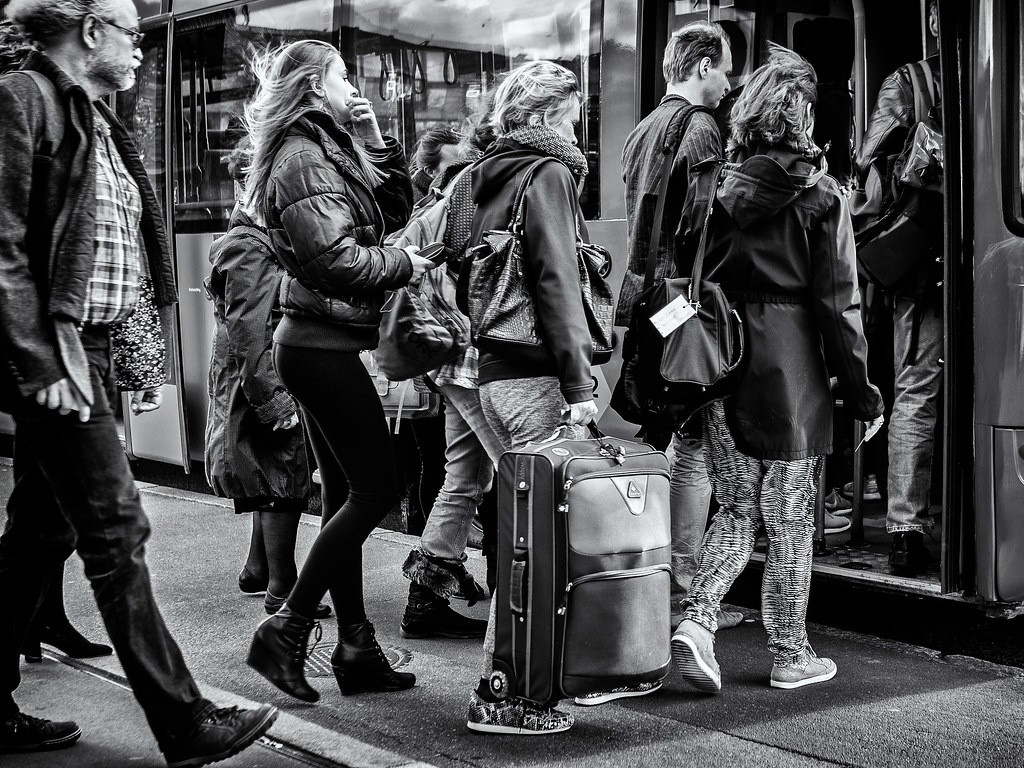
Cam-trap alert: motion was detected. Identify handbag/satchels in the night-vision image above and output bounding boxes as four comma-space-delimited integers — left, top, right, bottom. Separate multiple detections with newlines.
656, 278, 746, 393
847, 61, 945, 296
467, 156, 615, 365
361, 353, 441, 434
624, 284, 688, 421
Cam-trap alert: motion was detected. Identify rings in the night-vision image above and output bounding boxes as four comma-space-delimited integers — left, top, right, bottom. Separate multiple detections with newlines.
880, 422, 883, 423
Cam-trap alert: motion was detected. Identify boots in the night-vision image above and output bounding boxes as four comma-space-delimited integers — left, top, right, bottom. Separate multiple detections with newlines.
247, 603, 321, 703
332, 621, 416, 695
401, 549, 487, 639
24, 565, 112, 663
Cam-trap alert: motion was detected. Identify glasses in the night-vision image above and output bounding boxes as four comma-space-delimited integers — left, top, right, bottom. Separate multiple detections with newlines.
107, 21, 144, 48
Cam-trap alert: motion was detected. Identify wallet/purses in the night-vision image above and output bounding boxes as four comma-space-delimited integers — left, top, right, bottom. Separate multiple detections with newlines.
416, 242, 454, 267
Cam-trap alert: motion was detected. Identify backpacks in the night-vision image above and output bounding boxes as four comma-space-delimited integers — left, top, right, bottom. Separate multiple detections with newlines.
372, 160, 472, 381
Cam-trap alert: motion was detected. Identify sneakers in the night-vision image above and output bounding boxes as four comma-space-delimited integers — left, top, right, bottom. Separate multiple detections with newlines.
575, 681, 664, 706
0, 711, 81, 752
771, 644, 838, 689
467, 689, 575, 735
671, 619, 722, 691
165, 706, 277, 768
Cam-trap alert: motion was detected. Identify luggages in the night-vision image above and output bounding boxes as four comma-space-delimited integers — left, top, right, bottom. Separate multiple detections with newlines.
492, 412, 671, 704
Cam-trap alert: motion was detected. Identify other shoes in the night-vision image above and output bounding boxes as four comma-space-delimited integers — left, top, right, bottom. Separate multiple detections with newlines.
265, 587, 331, 619
822, 490, 852, 515
888, 531, 931, 578
239, 565, 268, 593
824, 509, 851, 533
716, 610, 744, 628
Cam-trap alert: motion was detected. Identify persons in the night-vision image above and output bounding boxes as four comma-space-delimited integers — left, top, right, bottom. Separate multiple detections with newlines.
672, 50, 887, 692
0, 0, 277, 768
401, 60, 661, 733
205, 40, 435, 700
619, 23, 740, 629
855, 1, 944, 563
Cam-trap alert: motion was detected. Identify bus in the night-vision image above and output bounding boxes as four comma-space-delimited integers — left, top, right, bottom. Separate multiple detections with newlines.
1, 0, 1024, 624
